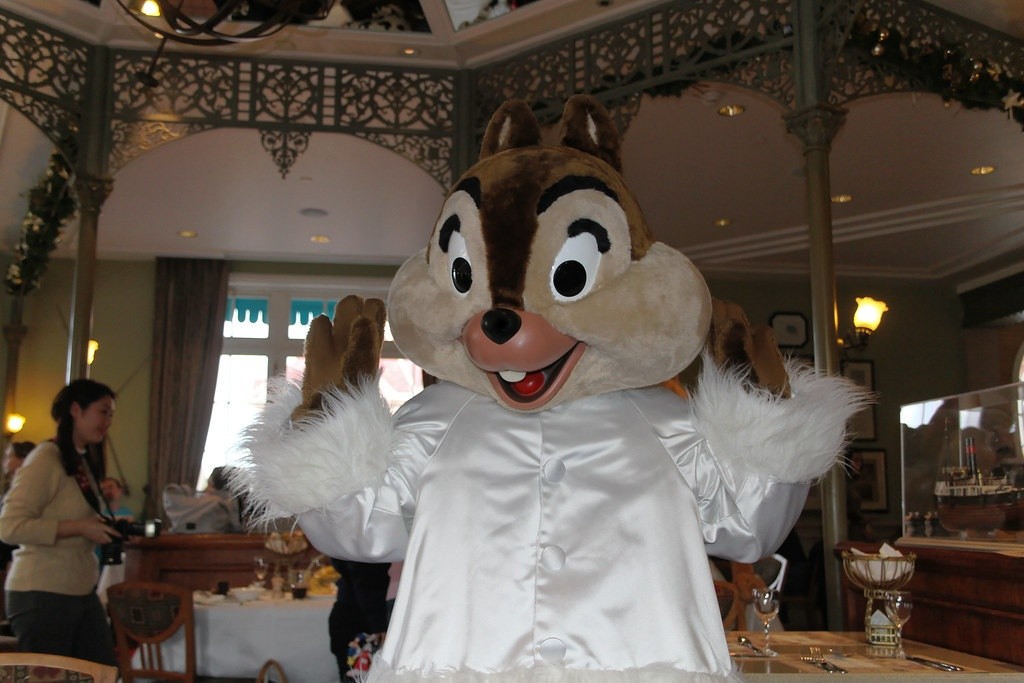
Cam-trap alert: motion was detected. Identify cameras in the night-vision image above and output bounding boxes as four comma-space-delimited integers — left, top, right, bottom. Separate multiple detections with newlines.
101, 519, 160, 565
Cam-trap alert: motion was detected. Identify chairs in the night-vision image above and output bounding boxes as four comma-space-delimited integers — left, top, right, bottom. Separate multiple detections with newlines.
107, 580, 198, 683
711, 540, 829, 630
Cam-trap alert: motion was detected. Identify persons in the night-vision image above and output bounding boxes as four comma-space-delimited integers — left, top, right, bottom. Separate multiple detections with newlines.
205, 465, 264, 530
328, 558, 404, 683
0, 378, 135, 679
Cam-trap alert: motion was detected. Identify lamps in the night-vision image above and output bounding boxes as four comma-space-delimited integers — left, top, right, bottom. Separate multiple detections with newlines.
4, 414, 26, 439
86, 340, 99, 364
841, 296, 889, 351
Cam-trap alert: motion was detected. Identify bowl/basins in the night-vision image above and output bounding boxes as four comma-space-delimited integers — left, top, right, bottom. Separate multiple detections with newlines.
229, 587, 264, 602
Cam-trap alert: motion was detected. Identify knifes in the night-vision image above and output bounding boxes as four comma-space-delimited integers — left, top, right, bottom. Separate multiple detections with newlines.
906, 657, 962, 671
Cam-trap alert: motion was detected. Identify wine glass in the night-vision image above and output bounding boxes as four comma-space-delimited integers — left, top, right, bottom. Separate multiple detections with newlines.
884, 591, 913, 666
752, 587, 780, 656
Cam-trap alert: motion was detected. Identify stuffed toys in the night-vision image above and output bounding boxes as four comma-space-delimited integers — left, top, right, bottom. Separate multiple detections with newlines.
223, 93, 879, 683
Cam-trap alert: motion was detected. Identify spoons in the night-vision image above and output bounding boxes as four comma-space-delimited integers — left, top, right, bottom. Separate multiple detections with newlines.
737, 636, 761, 656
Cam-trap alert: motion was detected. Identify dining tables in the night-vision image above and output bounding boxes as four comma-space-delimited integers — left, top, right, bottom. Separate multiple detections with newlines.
120, 593, 342, 683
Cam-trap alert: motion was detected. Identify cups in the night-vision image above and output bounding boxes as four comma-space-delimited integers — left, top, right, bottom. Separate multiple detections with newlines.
211, 572, 230, 596
254, 551, 269, 586
288, 570, 309, 599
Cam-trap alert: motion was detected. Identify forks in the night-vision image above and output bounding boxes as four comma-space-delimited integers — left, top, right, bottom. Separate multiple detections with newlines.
799, 646, 846, 673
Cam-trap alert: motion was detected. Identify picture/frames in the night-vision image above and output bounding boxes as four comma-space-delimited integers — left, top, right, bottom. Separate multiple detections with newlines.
844, 447, 889, 513
770, 312, 808, 347
839, 359, 877, 442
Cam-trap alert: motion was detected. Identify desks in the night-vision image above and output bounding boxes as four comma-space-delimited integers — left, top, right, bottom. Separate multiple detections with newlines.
724, 630, 1024, 683
123, 530, 336, 599
